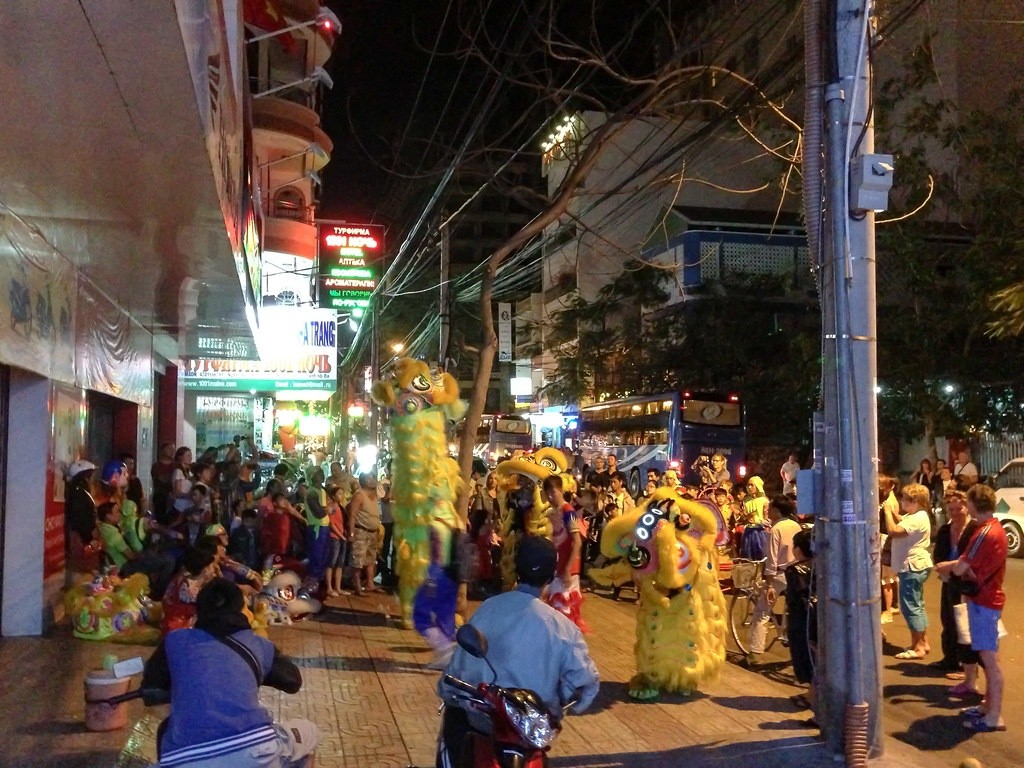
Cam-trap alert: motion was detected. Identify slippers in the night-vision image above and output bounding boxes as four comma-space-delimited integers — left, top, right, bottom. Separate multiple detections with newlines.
943, 684, 977, 694
961, 718, 1006, 731
959, 708, 985, 718
895, 648, 924, 659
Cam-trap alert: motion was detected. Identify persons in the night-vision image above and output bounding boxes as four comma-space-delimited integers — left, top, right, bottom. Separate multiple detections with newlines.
63, 435, 1009, 768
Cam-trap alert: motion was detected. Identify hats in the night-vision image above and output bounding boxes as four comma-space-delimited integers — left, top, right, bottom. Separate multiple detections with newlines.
307, 466, 320, 480
517, 535, 558, 574
748, 476, 764, 493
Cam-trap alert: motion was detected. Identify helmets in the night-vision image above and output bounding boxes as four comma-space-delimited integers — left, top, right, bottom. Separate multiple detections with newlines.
101, 459, 124, 482
69, 460, 95, 477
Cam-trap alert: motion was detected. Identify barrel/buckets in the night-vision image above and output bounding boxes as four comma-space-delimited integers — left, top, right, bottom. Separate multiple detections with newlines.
83, 667, 132, 732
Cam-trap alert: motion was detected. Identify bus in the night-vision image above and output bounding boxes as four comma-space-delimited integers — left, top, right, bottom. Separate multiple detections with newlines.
565, 391, 749, 502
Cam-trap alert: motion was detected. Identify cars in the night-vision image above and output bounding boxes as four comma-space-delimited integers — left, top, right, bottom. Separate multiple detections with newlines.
257, 450, 280, 471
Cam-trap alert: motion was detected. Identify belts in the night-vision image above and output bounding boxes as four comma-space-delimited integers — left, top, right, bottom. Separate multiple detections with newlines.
354, 524, 379, 533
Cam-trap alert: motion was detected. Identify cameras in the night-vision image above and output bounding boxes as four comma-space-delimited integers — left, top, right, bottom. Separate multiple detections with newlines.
702, 459, 709, 467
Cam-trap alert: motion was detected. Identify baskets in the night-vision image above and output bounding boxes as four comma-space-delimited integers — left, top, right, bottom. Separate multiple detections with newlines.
730, 563, 764, 588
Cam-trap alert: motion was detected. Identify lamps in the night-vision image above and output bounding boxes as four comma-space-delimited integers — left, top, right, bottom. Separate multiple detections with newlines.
256, 141, 326, 169
260, 169, 321, 197
244, 6, 343, 45
252, 64, 334, 99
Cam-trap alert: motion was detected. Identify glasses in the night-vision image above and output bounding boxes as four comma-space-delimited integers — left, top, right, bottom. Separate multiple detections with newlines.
218, 532, 227, 536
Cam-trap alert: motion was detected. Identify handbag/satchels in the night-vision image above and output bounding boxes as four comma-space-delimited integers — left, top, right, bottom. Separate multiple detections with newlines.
953, 603, 1008, 645
469, 490, 488, 540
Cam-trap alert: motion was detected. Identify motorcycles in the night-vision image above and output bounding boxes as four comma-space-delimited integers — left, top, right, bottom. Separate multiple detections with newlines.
436, 623, 583, 768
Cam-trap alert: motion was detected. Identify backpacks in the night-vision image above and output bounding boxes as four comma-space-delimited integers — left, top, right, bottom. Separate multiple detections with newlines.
433, 517, 482, 583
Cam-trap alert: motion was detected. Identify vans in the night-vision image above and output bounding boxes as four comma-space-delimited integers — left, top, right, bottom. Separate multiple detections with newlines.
984, 457, 1024, 558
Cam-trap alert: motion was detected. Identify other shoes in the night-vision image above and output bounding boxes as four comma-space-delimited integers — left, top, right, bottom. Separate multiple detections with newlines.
926, 660, 965, 672
336, 590, 351, 595
326, 590, 339, 597
880, 611, 893, 624
354, 591, 368, 597
890, 606, 900, 614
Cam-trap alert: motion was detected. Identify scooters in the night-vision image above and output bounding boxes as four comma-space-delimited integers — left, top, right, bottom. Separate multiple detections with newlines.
110, 686, 174, 766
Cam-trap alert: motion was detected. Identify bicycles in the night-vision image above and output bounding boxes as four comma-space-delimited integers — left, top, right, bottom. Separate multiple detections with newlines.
728, 557, 790, 656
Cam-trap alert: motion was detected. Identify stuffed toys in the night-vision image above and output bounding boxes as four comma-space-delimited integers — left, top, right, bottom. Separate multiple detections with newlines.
371, 357, 469, 629
248, 561, 322, 626
67, 570, 164, 643
587, 488, 728, 705
494, 447, 578, 593
